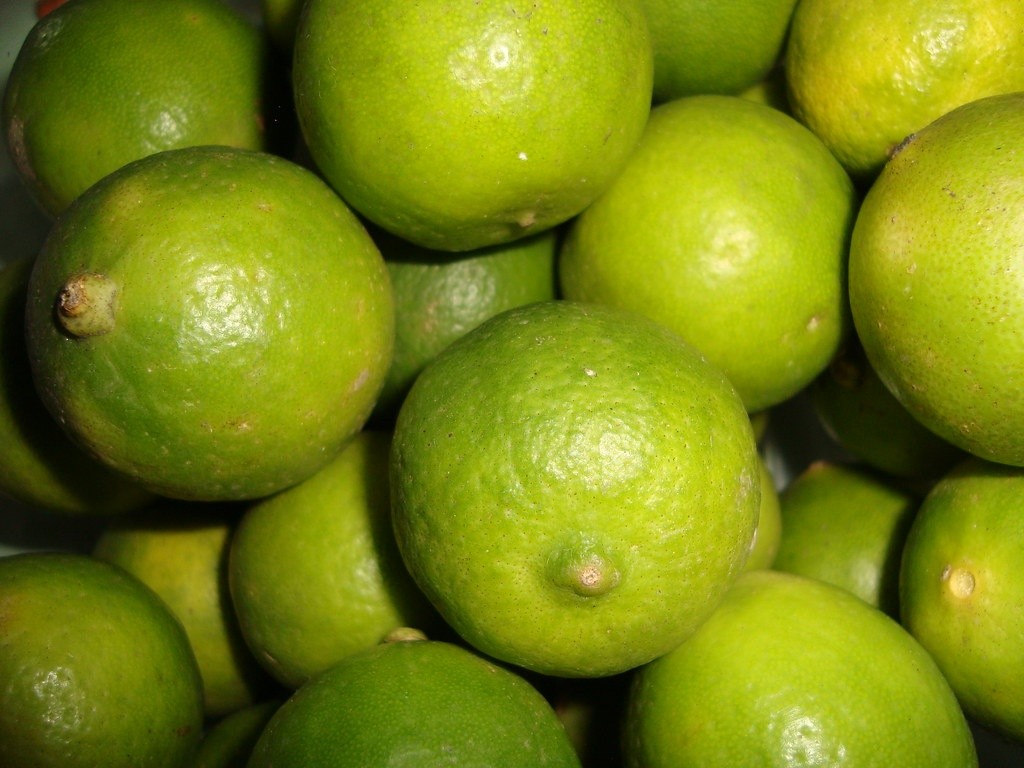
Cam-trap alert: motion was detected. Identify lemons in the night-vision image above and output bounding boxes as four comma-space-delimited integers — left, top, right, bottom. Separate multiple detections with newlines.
0, 0, 1024, 768
